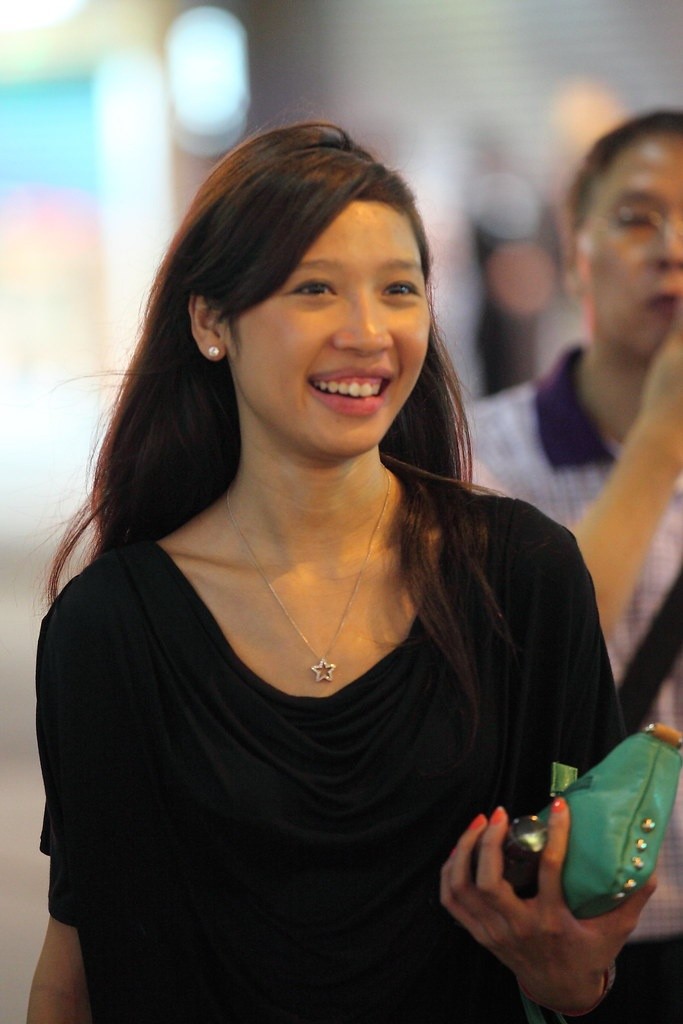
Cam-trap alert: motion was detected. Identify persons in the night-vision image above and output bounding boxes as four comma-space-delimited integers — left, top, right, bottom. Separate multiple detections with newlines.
447, 107, 683, 1021
459, 140, 565, 399
25, 122, 656, 1023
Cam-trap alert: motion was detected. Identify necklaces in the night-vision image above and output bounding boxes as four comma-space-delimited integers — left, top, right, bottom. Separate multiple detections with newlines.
225, 464, 391, 684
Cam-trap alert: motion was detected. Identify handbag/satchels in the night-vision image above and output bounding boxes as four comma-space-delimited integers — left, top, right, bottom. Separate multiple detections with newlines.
537, 722, 683, 921
